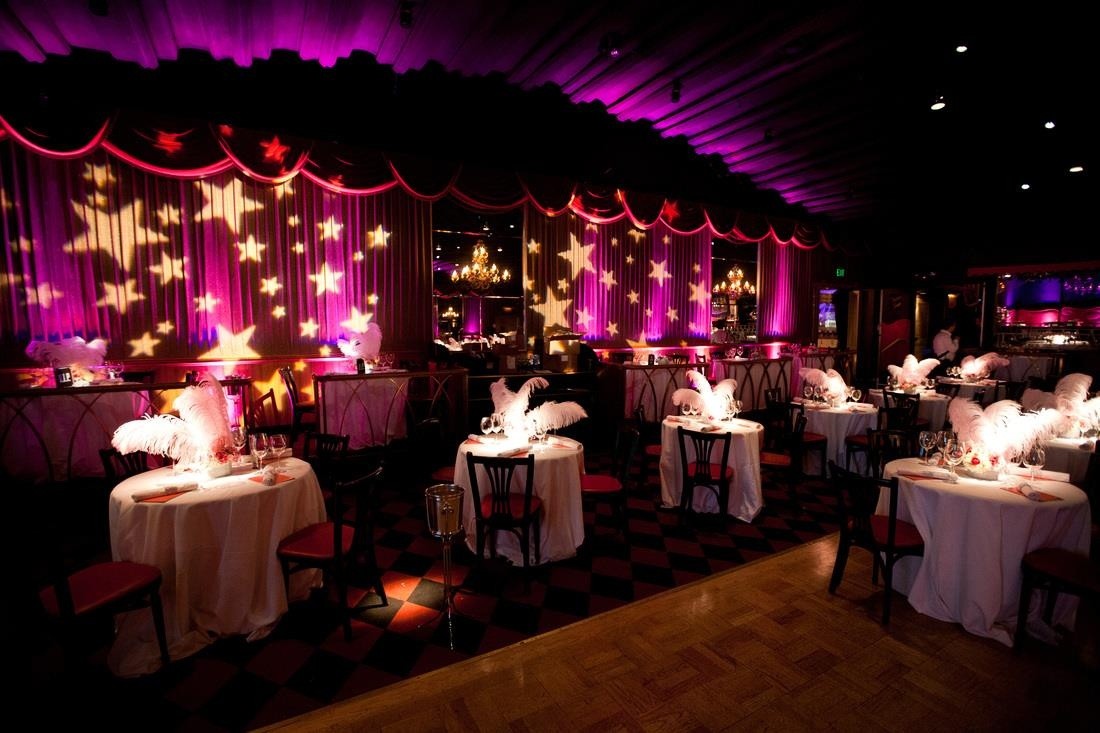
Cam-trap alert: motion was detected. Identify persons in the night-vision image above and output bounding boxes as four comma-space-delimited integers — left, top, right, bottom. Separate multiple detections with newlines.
933, 321, 959, 377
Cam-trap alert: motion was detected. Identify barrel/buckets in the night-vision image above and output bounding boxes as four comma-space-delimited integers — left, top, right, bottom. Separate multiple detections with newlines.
427, 484, 464, 532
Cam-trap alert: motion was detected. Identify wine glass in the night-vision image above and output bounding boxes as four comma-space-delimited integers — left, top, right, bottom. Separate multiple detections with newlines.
920, 431, 966, 473
804, 385, 861, 408
481, 413, 503, 439
724, 345, 744, 360
946, 366, 990, 382
232, 427, 288, 475
724, 324, 756, 343
682, 401, 743, 423
535, 431, 546, 446
1023, 449, 1046, 484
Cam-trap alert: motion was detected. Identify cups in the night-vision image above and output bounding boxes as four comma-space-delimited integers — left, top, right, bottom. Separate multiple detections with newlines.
366, 351, 394, 369
88, 360, 124, 377
888, 376, 936, 395
791, 340, 818, 354
750, 347, 761, 360
1010, 452, 1022, 466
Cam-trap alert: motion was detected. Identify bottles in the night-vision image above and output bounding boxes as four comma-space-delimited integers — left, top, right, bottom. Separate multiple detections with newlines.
53, 342, 73, 388
356, 341, 365, 372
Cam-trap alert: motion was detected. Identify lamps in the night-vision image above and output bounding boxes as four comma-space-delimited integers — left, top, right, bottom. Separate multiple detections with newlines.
450, 238, 512, 289
712, 262, 756, 301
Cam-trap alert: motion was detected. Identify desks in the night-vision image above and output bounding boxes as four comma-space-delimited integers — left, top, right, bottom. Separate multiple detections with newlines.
662, 413, 762, 524
0, 383, 160, 483
792, 396, 878, 473
109, 456, 328, 673
1031, 436, 1100, 474
876, 457, 1091, 651
936, 371, 1008, 410
328, 367, 407, 448
625, 358, 692, 421
712, 355, 792, 404
873, 385, 951, 436
451, 425, 584, 563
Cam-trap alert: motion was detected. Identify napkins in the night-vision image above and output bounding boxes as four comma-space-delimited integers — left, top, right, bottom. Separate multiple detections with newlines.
131, 480, 200, 503
469, 433, 496, 447
1015, 468, 1070, 482
261, 463, 277, 486
804, 404, 831, 409
498, 444, 532, 456
733, 418, 760, 430
794, 397, 813, 404
1018, 482, 1041, 500
897, 466, 950, 481
702, 424, 721, 432
549, 437, 582, 450
1076, 438, 1095, 451
667, 415, 691, 424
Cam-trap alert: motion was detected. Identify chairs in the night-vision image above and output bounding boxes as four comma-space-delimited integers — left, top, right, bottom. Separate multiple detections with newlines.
0, 353, 1100, 674
